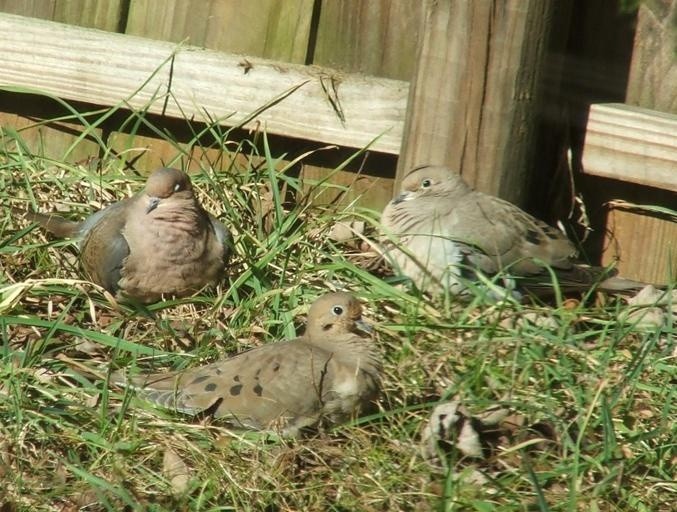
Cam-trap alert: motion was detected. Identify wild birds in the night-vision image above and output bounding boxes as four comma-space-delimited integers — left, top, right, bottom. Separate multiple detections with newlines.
378, 166, 669, 309
0, 167, 235, 306
57, 292, 385, 437
